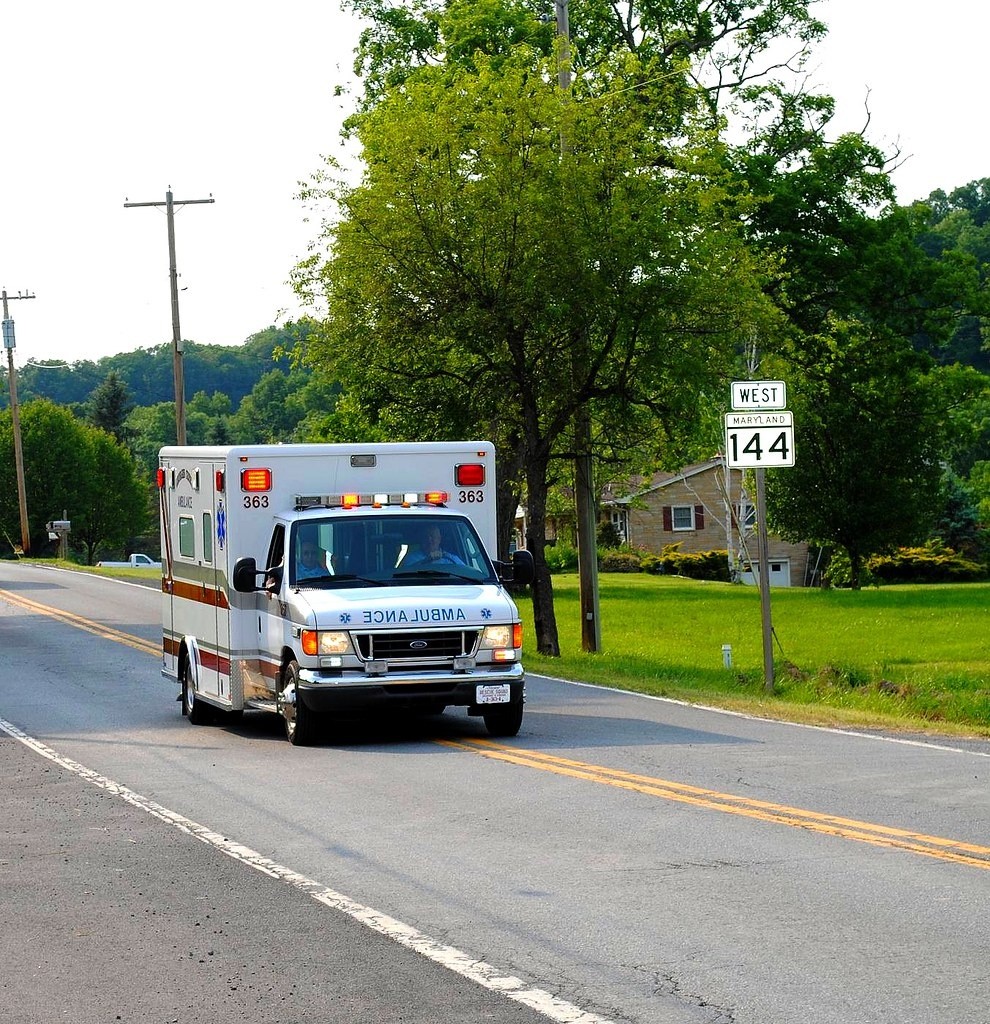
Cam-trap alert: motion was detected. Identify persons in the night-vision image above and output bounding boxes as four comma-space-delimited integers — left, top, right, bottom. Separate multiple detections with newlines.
403, 525, 465, 569
267, 539, 332, 586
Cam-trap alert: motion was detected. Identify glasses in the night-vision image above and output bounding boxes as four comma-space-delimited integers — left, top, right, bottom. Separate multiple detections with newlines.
301, 551, 318, 556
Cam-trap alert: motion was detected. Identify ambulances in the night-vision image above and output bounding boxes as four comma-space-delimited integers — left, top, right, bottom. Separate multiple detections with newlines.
157, 442, 536, 747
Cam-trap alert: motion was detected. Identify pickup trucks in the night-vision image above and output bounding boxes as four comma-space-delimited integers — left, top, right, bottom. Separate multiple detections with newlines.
97, 554, 162, 568
